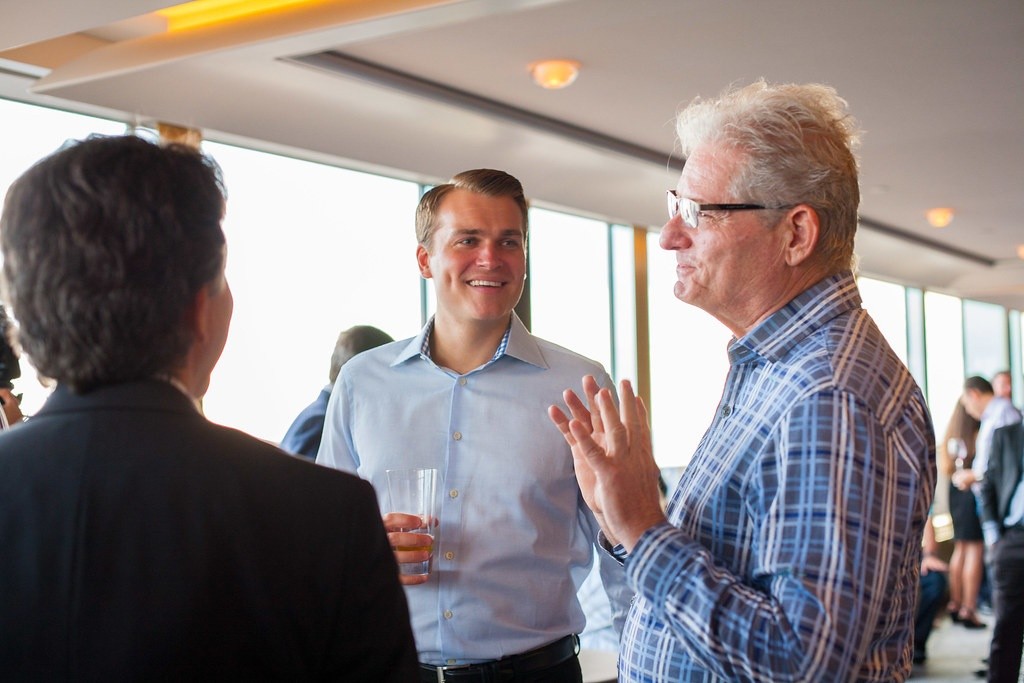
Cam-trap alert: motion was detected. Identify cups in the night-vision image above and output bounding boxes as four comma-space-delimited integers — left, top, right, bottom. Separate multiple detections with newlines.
384, 466, 437, 580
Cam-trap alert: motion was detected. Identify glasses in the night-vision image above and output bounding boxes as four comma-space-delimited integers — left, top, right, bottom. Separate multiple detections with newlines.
666, 187, 793, 228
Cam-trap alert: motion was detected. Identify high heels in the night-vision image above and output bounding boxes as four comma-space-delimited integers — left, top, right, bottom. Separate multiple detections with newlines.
948, 600, 986, 629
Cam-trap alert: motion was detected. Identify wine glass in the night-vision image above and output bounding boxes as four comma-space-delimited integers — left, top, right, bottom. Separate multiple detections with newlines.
947, 437, 968, 474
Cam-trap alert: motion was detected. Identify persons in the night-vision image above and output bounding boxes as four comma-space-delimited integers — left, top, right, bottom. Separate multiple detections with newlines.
0, 306, 30, 430
314, 167, 636, 683
910, 502, 950, 674
545, 80, 935, 683
282, 322, 399, 466
944, 363, 1024, 682
0, 131, 431, 683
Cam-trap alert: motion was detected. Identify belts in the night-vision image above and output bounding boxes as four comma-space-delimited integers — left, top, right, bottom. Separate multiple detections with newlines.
419, 635, 578, 683
